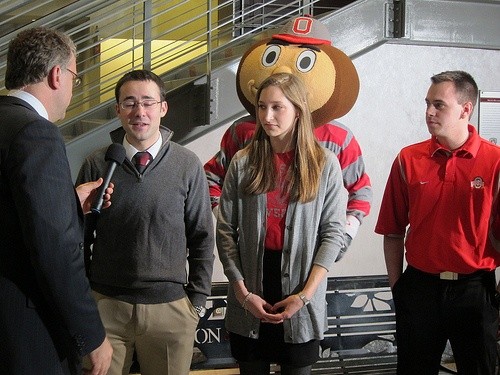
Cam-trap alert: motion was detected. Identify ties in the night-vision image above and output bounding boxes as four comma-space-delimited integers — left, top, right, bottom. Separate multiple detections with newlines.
134, 152, 151, 174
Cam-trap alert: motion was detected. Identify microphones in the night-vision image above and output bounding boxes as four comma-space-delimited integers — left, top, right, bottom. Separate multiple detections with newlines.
92, 142, 126, 214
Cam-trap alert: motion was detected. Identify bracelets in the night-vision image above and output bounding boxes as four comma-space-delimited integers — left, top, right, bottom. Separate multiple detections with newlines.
235, 287, 247, 295
297, 292, 309, 306
242, 292, 252, 307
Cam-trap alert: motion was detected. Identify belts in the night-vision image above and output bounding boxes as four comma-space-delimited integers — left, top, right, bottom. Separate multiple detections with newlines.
409, 266, 478, 280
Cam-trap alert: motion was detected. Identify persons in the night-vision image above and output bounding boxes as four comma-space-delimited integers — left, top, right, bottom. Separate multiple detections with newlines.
0, 27, 113, 375
374, 71, 500, 375
215, 73, 348, 375
74, 70, 216, 375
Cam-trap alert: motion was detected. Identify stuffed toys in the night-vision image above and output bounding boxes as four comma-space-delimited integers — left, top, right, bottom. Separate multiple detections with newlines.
203, 14, 373, 260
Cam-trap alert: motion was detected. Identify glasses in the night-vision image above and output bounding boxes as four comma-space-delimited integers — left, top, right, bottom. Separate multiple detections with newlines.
119, 100, 162, 110
64, 67, 81, 88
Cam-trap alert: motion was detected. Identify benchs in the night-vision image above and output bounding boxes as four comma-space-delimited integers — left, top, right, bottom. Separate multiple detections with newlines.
186, 274, 456, 375
